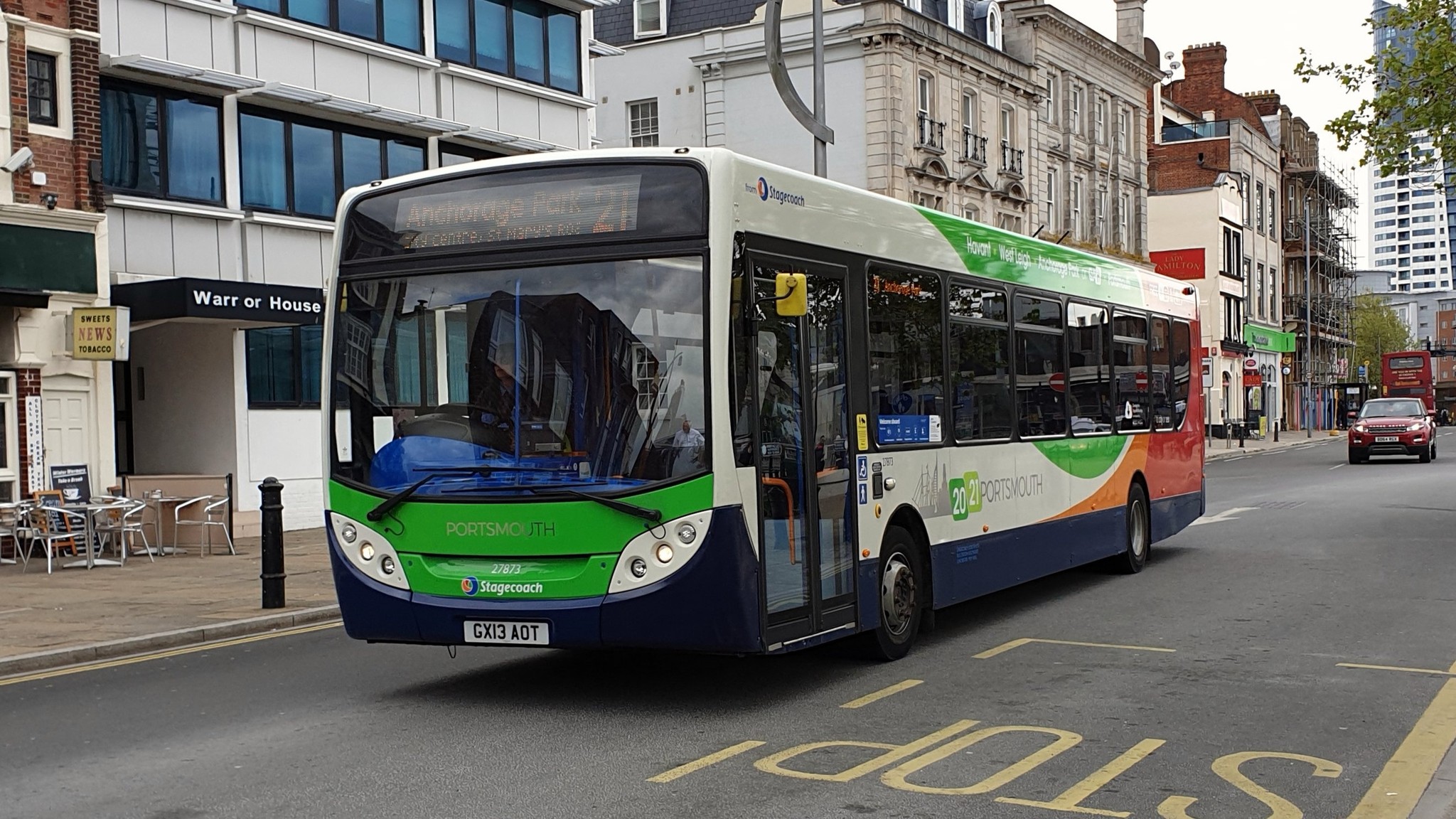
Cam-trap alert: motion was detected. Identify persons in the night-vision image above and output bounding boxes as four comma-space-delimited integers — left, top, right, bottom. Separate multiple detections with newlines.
1435, 407, 1449, 426
469, 343, 543, 457
774, 410, 846, 473
1328, 394, 1356, 430
672, 420, 704, 462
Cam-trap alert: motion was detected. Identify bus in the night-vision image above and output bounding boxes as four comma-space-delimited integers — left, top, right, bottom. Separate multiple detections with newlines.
312, 143, 1209, 662
1381, 350, 1434, 411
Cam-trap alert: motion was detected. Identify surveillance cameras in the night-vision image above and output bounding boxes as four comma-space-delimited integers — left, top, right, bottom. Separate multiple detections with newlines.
0, 146, 33, 173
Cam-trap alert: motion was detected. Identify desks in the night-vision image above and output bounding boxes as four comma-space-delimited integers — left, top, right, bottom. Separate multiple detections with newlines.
60, 503, 135, 570
134, 496, 200, 557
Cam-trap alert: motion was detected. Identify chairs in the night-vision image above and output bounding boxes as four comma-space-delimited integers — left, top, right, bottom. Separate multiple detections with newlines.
1224, 418, 1260, 440
0, 490, 238, 575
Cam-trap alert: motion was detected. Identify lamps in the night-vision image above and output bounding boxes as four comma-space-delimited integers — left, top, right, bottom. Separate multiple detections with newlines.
1235, 345, 1257, 357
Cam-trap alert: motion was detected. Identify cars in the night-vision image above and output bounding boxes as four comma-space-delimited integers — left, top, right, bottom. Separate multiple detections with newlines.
1346, 397, 1438, 463
